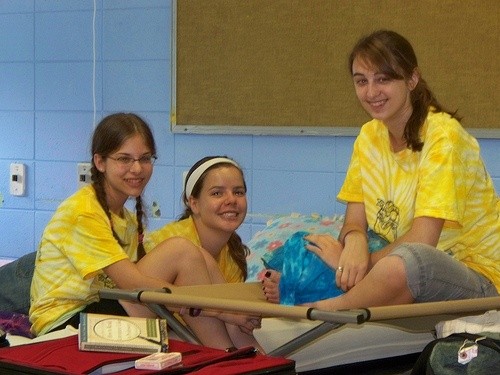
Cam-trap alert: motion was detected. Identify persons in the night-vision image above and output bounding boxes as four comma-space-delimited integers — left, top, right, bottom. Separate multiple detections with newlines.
298, 29, 500, 311
145, 154, 280, 342
28, 112, 268, 355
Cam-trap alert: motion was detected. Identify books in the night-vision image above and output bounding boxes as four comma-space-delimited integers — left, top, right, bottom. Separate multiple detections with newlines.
77, 311, 168, 353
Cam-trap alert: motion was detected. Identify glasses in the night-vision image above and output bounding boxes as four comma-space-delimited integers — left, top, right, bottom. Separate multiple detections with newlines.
105, 154, 158, 166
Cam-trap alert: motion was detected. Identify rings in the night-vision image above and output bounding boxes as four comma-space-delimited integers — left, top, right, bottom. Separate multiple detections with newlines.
338, 267, 343, 271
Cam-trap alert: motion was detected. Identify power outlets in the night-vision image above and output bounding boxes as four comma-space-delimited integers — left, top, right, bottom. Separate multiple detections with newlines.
9, 162, 26, 197
76, 162, 93, 192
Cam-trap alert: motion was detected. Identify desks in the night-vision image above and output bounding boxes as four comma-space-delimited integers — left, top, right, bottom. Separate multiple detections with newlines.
0, 324, 297, 375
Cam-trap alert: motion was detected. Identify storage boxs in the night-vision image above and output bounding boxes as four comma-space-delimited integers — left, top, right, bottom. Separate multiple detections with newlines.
133, 352, 182, 371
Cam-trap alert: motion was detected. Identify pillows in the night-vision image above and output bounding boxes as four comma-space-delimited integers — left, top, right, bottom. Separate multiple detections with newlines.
243, 213, 346, 285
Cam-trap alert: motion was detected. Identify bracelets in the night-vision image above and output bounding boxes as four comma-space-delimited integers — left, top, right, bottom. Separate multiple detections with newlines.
179, 306, 202, 316
